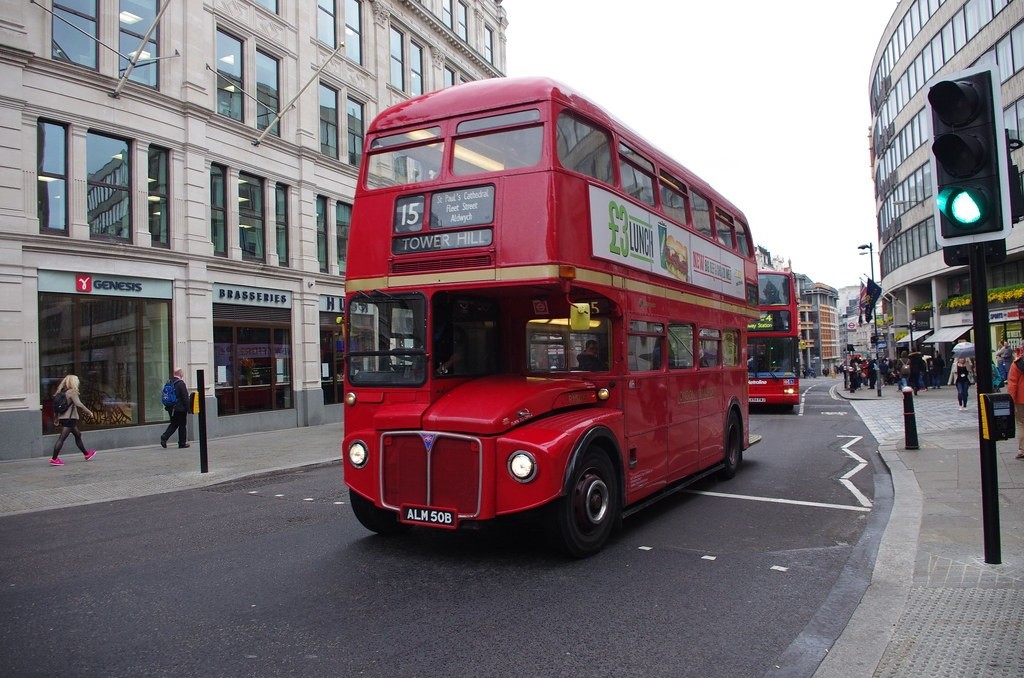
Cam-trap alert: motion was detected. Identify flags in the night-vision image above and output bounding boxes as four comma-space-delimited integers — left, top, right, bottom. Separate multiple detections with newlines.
858, 278, 882, 328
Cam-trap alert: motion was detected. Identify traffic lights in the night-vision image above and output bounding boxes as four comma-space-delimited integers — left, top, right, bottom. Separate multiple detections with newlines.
927, 64, 1012, 246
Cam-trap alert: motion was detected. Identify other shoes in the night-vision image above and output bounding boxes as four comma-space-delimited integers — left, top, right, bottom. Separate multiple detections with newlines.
161, 434, 167, 448
959, 406, 962, 411
963, 407, 967, 410
179, 444, 190, 448
1014, 453, 1024, 460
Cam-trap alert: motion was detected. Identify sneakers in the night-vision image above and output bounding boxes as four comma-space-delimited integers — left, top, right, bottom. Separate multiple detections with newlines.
50, 457, 64, 465
85, 449, 96, 460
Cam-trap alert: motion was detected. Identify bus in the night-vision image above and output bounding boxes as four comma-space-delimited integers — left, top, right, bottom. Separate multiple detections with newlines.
337, 74, 763, 560
747, 270, 807, 410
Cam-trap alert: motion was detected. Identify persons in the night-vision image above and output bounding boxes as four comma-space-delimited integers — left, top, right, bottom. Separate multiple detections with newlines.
49, 375, 97, 466
802, 368, 807, 378
576, 340, 598, 368
948, 340, 1013, 411
848, 348, 945, 395
652, 336, 675, 369
1007, 340, 1024, 458
432, 302, 469, 372
161, 369, 190, 448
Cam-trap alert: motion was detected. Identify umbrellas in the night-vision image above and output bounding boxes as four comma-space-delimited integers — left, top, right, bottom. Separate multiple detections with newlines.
952, 351, 975, 358
952, 342, 975, 352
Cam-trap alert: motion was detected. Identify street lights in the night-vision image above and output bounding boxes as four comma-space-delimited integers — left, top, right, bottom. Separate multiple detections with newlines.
857, 243, 881, 397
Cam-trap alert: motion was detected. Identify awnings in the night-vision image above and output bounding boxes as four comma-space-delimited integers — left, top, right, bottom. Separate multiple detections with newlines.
921, 326, 973, 342
897, 329, 932, 342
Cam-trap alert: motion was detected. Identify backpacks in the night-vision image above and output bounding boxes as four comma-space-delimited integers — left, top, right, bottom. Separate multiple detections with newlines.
899, 358, 910, 378
162, 379, 181, 406
53, 388, 75, 414
